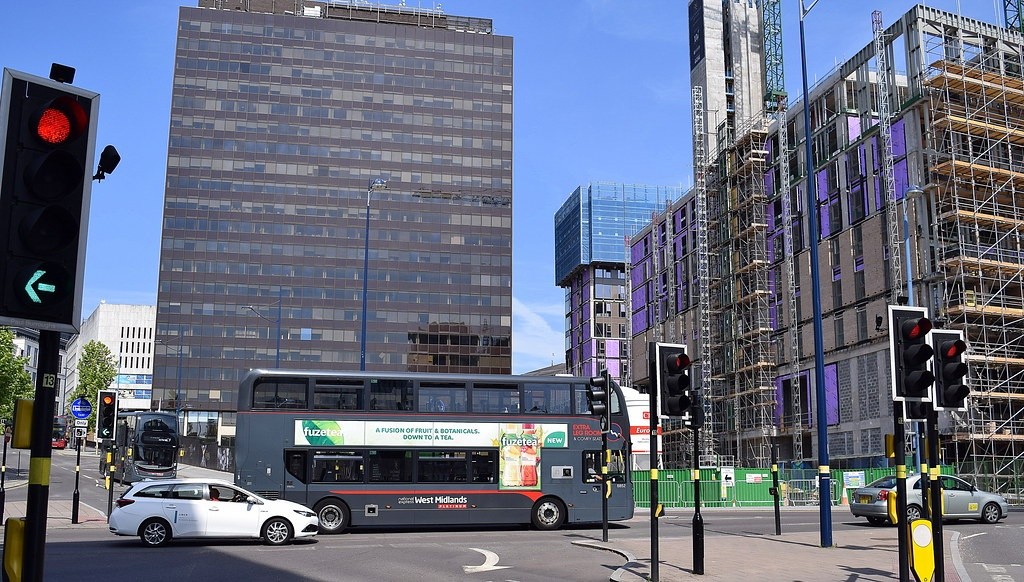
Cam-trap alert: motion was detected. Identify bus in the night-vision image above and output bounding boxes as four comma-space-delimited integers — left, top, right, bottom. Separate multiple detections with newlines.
99, 412, 184, 483
233, 368, 635, 536
312, 455, 493, 482
52, 416, 67, 449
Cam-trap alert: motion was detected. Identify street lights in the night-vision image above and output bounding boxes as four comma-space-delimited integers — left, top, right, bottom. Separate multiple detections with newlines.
241, 286, 282, 367
154, 327, 183, 414
361, 178, 389, 371
903, 187, 923, 473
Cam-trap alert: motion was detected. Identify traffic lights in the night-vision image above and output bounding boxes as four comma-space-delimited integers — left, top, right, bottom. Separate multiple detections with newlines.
929, 329, 971, 411
0, 67, 101, 335
589, 368, 610, 433
888, 305, 936, 402
96, 390, 118, 440
656, 342, 691, 420
902, 401, 927, 422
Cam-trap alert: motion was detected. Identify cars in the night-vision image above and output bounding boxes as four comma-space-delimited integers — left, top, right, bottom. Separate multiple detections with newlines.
108, 478, 319, 548
850, 474, 1008, 528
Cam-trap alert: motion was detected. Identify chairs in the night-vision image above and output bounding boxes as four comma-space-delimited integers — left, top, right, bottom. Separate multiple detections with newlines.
369, 397, 377, 410
396, 401, 412, 410
